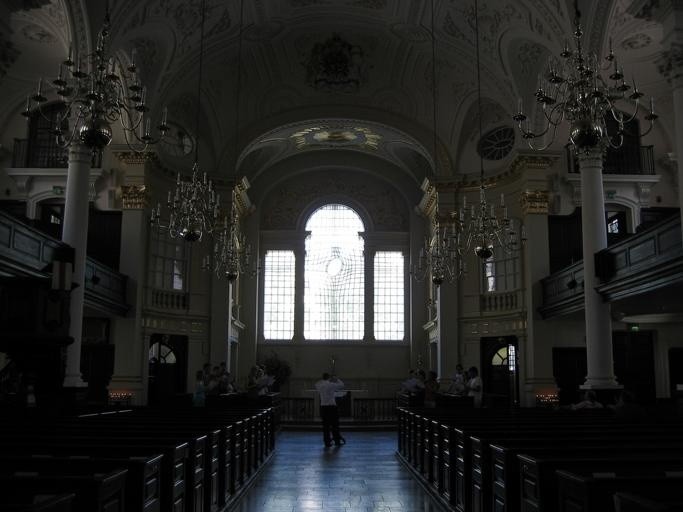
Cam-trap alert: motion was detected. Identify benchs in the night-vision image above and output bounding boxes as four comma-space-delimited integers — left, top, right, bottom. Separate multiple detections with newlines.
0, 406, 274, 512
395, 403, 682, 512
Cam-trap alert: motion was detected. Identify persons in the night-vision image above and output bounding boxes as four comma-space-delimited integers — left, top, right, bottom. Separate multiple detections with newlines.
192, 359, 265, 403
314, 372, 345, 447
400, 363, 484, 407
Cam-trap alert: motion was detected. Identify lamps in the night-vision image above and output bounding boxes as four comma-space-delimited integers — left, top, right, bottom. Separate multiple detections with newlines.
403, 0, 464, 286
148, 0, 220, 246
511, 0, 655, 159
22, 0, 170, 165
457, 0, 525, 258
203, 0, 262, 284
